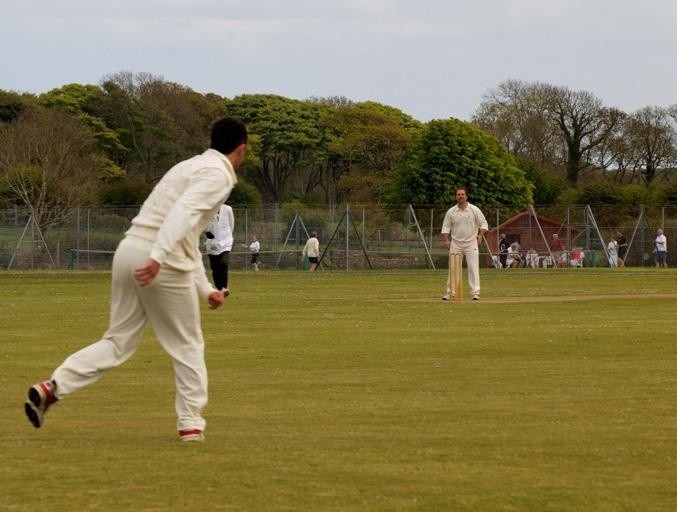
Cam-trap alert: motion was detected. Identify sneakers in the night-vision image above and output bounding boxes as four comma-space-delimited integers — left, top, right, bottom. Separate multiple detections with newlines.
221, 287, 230, 297
25, 380, 58, 428
442, 295, 454, 300
177, 429, 205, 443
473, 295, 480, 300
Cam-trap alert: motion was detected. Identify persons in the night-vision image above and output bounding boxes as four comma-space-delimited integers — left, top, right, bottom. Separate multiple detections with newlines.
615, 229, 628, 267
438, 185, 489, 300
496, 233, 584, 269
653, 228, 669, 269
606, 234, 618, 267
203, 203, 235, 298
301, 230, 320, 272
23, 115, 250, 445
249, 234, 260, 273
